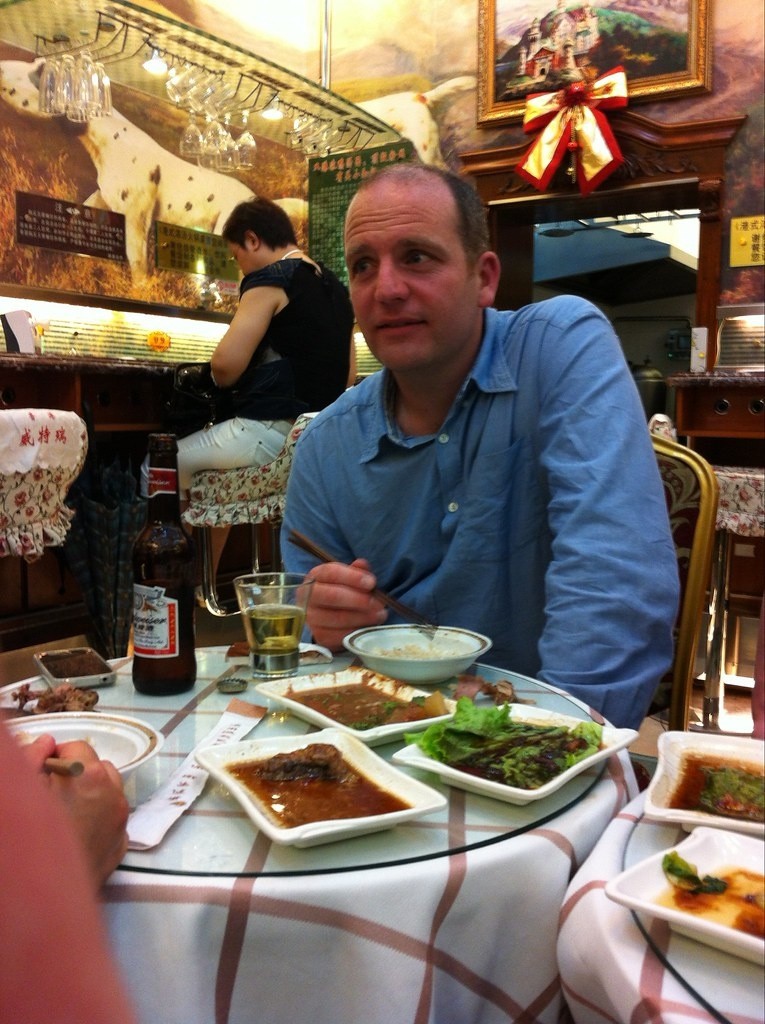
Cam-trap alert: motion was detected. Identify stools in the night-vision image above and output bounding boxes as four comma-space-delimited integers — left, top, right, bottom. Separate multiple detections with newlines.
648, 465, 765, 737
185, 407, 320, 617
0, 408, 88, 650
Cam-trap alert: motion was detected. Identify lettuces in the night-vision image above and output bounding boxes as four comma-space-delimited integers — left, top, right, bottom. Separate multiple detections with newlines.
401, 696, 603, 788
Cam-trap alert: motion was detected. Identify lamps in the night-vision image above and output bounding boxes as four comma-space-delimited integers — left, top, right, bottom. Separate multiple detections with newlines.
259, 89, 285, 120
143, 46, 167, 75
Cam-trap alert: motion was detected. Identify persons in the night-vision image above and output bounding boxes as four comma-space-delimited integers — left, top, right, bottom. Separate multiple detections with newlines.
282, 162, 681, 736
138, 196, 356, 608
0, 720, 135, 1024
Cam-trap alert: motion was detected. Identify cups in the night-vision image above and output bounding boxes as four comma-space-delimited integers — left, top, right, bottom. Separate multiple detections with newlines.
232, 572, 315, 683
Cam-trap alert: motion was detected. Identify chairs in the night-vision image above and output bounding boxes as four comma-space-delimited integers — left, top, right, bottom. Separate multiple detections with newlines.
0, 633, 108, 689
644, 429, 720, 759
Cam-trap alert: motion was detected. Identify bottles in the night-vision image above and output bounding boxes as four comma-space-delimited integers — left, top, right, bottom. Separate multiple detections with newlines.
131, 429, 198, 697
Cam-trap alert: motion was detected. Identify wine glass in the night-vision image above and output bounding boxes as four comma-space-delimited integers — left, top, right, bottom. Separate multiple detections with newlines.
35, 34, 341, 171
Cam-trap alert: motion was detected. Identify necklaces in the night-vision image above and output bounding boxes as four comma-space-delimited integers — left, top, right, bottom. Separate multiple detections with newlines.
282, 250, 301, 260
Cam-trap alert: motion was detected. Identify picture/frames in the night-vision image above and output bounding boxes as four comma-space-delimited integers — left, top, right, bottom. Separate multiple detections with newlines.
474, 0, 713, 130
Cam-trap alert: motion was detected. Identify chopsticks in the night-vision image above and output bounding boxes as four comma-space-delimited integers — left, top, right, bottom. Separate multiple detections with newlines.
44, 758, 84, 775
288, 530, 440, 628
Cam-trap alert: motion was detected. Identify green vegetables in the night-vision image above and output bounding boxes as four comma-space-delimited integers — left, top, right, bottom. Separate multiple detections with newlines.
349, 695, 427, 731
695, 765, 765, 818
663, 852, 728, 895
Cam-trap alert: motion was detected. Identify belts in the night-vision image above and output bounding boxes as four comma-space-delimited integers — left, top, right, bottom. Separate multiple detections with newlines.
261, 420, 293, 436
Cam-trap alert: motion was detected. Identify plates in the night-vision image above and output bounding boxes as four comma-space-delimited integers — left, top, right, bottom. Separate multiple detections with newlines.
643, 730, 765, 840
602, 825, 765, 967
194, 726, 449, 849
390, 701, 641, 807
254, 665, 458, 749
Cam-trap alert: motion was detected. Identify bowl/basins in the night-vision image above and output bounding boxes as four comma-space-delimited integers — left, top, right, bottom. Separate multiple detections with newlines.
342, 623, 493, 685
1, 710, 165, 786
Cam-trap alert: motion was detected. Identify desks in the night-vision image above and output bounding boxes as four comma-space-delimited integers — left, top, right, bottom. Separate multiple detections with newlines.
0, 642, 639, 1024
553, 783, 765, 1024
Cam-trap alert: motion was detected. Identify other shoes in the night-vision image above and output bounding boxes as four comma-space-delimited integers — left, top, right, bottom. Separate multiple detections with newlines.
194, 585, 221, 610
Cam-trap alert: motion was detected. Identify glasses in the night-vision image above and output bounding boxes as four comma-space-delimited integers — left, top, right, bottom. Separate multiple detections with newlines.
226, 246, 241, 263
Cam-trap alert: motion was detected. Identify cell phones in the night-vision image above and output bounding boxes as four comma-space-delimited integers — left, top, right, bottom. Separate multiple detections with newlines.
34, 646, 116, 689
